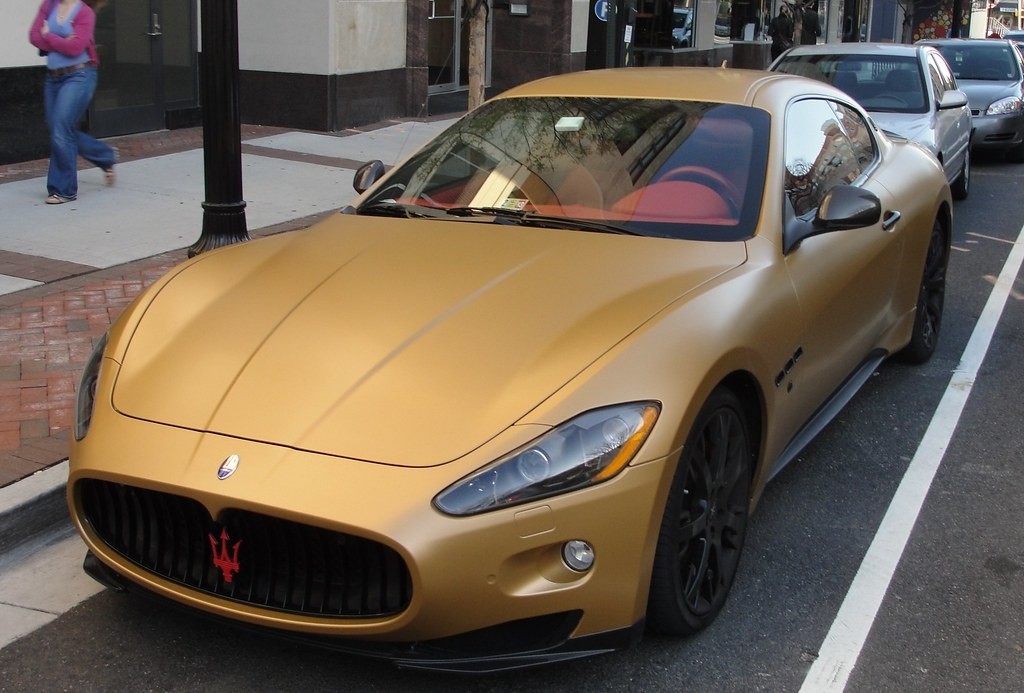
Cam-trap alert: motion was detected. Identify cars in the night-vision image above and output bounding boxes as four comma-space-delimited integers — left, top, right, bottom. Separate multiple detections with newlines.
988, 2, 1018, 25
1003, 31, 1024, 54
765, 43, 973, 199
715, 17, 731, 37
898, 38, 1024, 163
671, 9, 693, 49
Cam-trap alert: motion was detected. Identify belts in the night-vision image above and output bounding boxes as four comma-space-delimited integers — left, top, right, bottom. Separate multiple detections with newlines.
51, 62, 92, 76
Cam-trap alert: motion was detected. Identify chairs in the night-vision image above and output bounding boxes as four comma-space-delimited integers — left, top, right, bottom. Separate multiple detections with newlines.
881, 69, 922, 107
647, 115, 754, 207
829, 71, 858, 100
513, 111, 635, 210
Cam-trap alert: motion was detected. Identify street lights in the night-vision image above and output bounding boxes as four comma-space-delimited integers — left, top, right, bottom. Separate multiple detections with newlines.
986, 0, 994, 38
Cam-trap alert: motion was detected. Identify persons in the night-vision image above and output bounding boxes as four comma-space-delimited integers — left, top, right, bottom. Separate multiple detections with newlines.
988, 28, 1001, 39
29, 0, 120, 204
800, 0, 823, 45
768, 6, 794, 64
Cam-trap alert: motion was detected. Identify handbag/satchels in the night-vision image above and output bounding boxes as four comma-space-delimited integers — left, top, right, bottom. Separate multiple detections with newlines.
781, 39, 793, 51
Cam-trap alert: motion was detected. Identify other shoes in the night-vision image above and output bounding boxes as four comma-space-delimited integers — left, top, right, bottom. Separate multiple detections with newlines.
106, 165, 115, 185
46, 195, 71, 204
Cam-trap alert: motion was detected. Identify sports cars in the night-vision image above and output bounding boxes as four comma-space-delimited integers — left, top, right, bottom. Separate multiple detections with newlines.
65, 67, 954, 674
